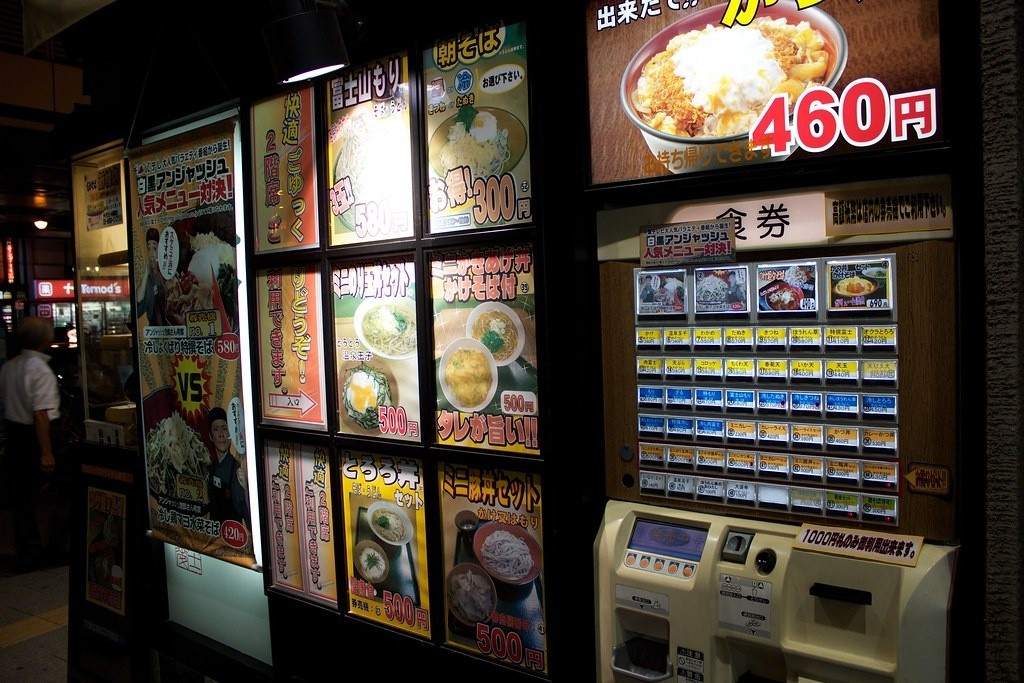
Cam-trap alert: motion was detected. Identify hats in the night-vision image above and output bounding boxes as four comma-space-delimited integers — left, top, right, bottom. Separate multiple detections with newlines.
206, 407, 228, 426
145, 227, 159, 243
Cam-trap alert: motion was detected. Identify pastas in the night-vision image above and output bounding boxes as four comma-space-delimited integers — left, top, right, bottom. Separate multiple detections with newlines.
437, 110, 513, 184
481, 529, 536, 581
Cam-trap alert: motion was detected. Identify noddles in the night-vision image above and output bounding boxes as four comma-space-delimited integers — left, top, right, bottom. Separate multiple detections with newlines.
363, 304, 417, 353
373, 507, 407, 543
474, 310, 518, 363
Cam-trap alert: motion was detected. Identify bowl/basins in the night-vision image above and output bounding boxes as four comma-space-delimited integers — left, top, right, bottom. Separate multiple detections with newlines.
861, 266, 887, 289
831, 275, 880, 302
333, 100, 541, 629
617, 0, 849, 176
154, 202, 245, 337
142, 386, 224, 515
763, 282, 806, 313
653, 291, 676, 306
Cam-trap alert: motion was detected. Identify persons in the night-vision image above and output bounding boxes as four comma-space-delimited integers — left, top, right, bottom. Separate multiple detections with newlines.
639, 277, 671, 303
137, 227, 166, 326
91, 314, 101, 338
726, 271, 746, 303
1, 318, 61, 567
204, 407, 252, 538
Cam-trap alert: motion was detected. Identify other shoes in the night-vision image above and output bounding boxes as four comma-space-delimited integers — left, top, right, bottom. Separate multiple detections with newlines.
22, 552, 67, 573
